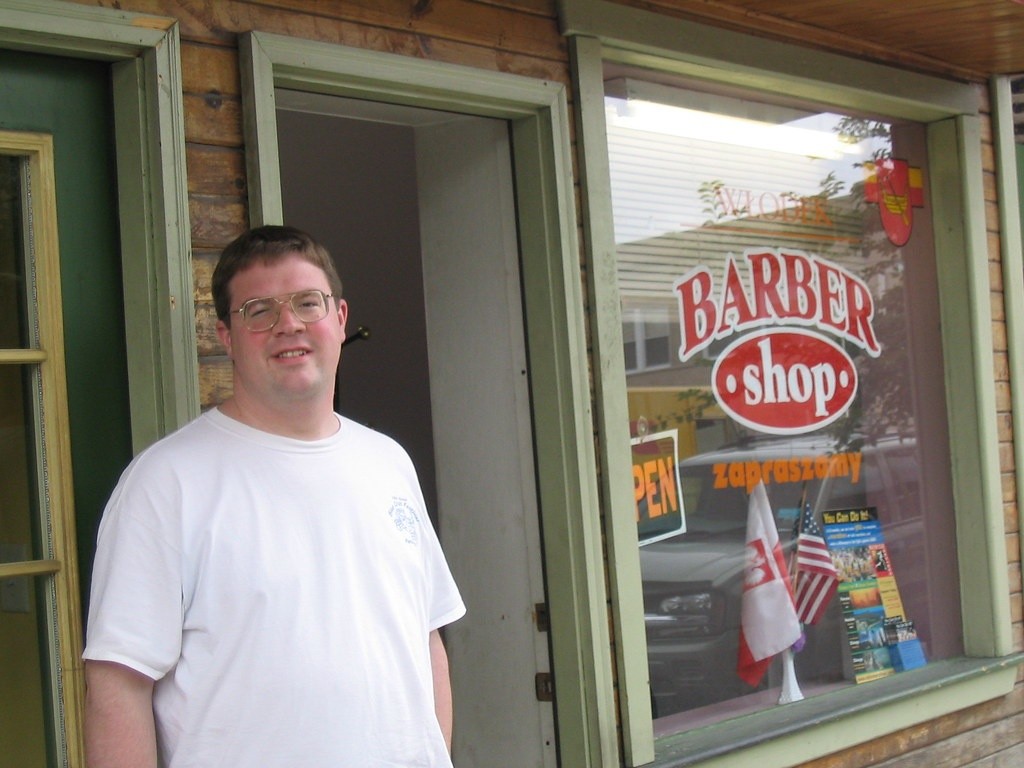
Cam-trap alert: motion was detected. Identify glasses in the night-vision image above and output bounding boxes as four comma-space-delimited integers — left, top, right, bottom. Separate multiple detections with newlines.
227, 289, 334, 333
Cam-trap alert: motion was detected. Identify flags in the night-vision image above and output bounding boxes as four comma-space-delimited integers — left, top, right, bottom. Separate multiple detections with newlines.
737, 476, 839, 688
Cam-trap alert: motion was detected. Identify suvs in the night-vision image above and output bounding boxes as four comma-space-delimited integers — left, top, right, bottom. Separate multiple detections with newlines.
637, 432, 927, 714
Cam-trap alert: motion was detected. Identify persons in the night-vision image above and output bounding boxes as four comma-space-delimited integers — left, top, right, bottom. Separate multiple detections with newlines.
81, 227, 466, 767
